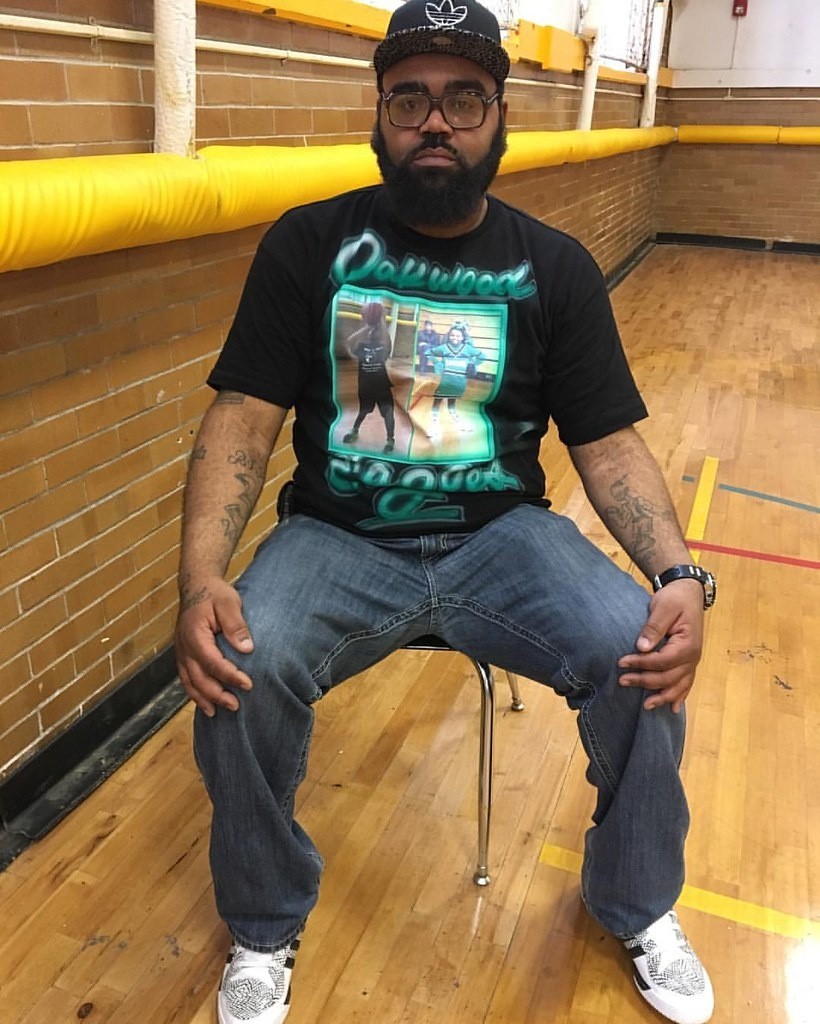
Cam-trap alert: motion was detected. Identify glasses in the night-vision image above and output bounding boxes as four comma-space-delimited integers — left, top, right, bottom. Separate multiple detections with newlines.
380, 91, 500, 130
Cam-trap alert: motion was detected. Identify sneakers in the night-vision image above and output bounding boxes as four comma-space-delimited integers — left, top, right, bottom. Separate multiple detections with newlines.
217, 911, 311, 1024
620, 905, 715, 1024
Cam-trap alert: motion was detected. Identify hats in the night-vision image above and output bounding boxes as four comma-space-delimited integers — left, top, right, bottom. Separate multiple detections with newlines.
373, 0, 510, 85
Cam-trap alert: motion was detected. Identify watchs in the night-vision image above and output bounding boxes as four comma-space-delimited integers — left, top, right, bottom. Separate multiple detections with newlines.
653, 564, 717, 611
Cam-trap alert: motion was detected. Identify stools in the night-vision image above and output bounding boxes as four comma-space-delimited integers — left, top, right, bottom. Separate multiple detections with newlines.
400, 634, 524, 886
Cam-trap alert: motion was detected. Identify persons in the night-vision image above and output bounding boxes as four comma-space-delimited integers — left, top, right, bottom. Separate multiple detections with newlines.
417, 320, 485, 428
342, 313, 395, 452
173, 0, 715, 1024
